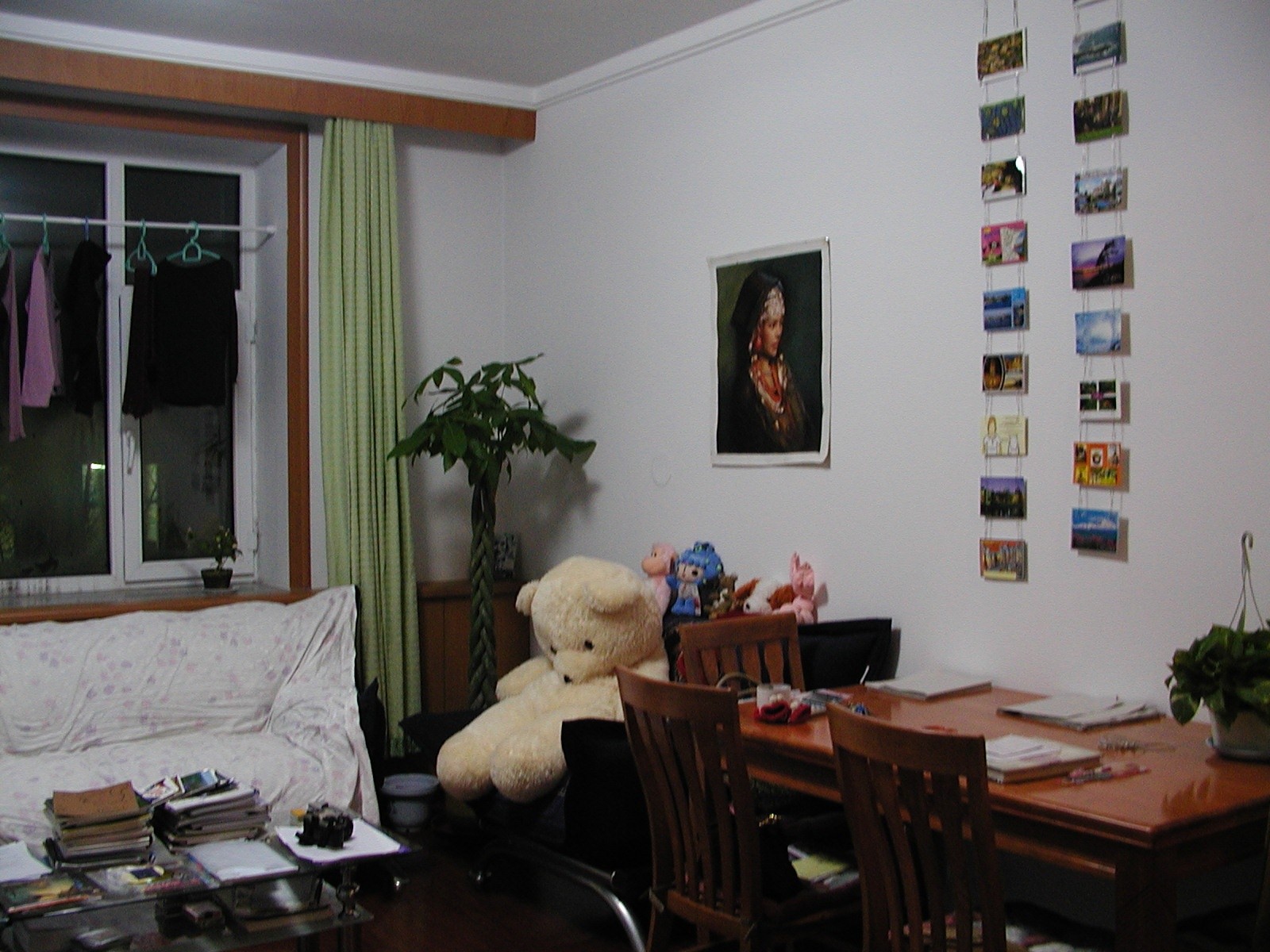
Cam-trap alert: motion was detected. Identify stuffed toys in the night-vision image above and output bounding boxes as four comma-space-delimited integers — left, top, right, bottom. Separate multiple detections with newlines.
702, 550, 818, 626
433, 555, 670, 804
641, 540, 680, 614
665, 538, 724, 615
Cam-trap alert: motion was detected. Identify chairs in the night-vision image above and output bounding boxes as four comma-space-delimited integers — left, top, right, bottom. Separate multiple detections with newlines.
614, 664, 865, 952
823, 699, 1098, 952
678, 611, 807, 710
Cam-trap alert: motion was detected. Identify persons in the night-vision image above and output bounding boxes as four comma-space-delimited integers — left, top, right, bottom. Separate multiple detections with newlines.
727, 268, 820, 452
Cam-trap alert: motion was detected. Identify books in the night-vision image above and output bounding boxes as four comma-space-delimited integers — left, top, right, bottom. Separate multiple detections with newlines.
1, 767, 334, 952
871, 670, 1159, 784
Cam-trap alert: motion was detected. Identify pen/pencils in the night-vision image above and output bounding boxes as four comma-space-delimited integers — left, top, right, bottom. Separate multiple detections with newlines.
1069, 762, 1152, 785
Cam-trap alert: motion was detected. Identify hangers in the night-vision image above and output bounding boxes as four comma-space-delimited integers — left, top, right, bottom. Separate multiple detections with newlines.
84, 217, 89, 242
126, 218, 158, 275
0, 212, 12, 249
41, 212, 50, 255
167, 220, 221, 265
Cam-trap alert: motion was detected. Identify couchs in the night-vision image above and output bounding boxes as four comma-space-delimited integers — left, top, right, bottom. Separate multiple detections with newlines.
1, 582, 376, 834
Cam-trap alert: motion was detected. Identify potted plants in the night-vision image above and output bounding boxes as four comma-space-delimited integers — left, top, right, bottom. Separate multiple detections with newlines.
1164, 528, 1270, 762
386, 347, 593, 853
186, 525, 244, 588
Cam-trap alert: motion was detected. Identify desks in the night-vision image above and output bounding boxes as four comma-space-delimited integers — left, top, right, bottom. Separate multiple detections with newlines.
700, 674, 1270, 952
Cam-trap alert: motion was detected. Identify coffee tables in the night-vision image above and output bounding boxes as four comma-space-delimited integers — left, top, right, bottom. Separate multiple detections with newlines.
0, 801, 423, 952
